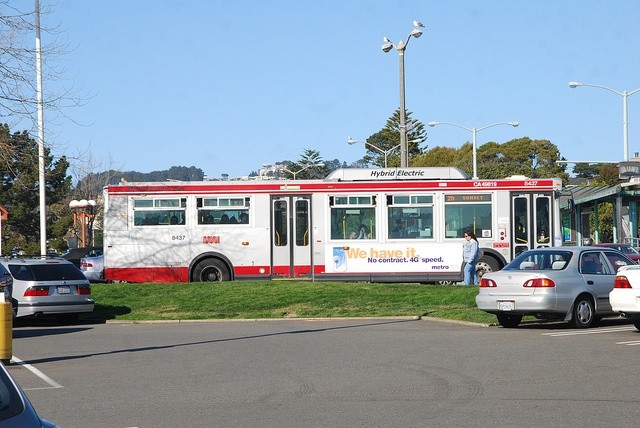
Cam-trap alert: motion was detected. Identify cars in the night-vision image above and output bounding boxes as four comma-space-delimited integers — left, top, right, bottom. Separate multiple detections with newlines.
0, 255, 95, 320
560, 243, 640, 273
476, 246, 638, 328
609, 265, 640, 330
61, 247, 103, 268
80, 248, 104, 281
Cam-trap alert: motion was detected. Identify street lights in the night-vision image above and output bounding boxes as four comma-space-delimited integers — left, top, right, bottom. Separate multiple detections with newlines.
568, 82, 639, 162
348, 139, 423, 167
429, 121, 519, 179
263, 164, 325, 180
382, 21, 425, 166
69, 199, 96, 248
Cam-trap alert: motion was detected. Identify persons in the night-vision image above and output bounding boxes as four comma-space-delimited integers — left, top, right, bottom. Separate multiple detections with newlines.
358, 224, 371, 239
135, 213, 185, 224
349, 230, 357, 239
204, 214, 248, 223
390, 218, 408, 239
462, 231, 479, 286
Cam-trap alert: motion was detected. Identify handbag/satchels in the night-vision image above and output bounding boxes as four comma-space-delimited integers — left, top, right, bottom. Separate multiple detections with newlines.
478, 247, 484, 261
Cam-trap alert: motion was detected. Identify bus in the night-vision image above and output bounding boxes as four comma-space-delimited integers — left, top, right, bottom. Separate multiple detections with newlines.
103, 167, 573, 282
